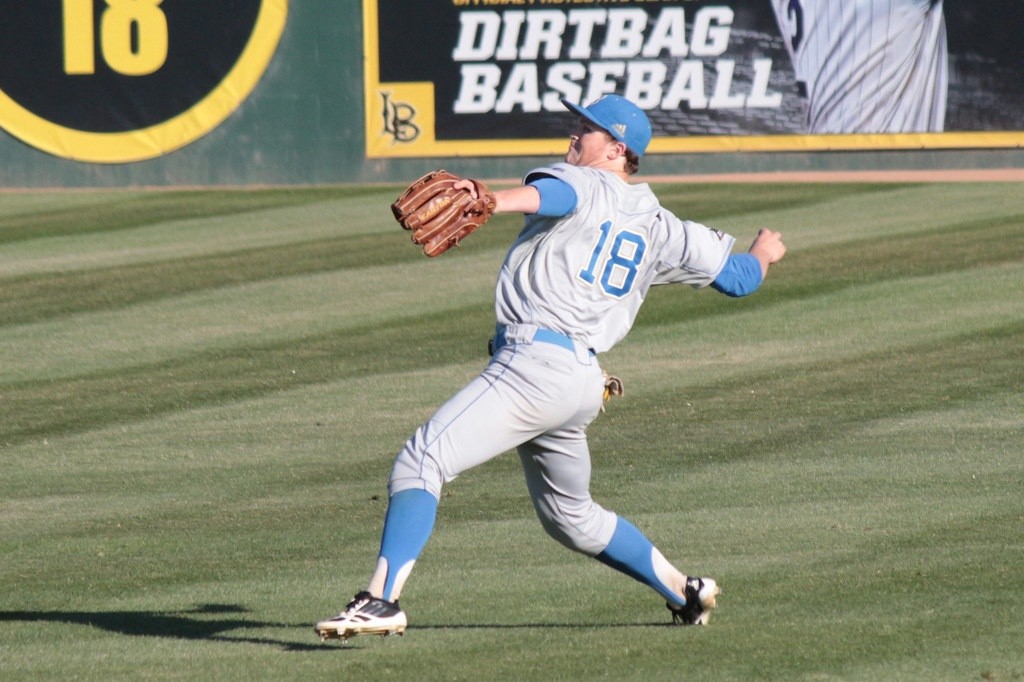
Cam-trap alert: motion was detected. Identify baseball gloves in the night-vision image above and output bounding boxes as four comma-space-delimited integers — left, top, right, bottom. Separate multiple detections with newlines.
390, 169, 497, 258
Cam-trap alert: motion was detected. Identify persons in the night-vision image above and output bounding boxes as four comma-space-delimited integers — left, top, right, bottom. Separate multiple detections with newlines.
316, 92, 788, 638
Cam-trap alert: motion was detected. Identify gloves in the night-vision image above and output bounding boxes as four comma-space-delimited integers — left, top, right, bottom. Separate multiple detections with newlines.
602, 370, 624, 401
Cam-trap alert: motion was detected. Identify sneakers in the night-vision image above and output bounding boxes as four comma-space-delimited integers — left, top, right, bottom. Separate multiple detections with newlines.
316, 590, 407, 644
666, 577, 722, 626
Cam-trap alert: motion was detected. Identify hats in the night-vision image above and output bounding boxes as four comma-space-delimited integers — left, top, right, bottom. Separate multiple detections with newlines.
561, 94, 652, 157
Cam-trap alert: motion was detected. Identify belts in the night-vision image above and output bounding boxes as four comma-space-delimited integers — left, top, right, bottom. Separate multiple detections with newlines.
488, 326, 594, 357
